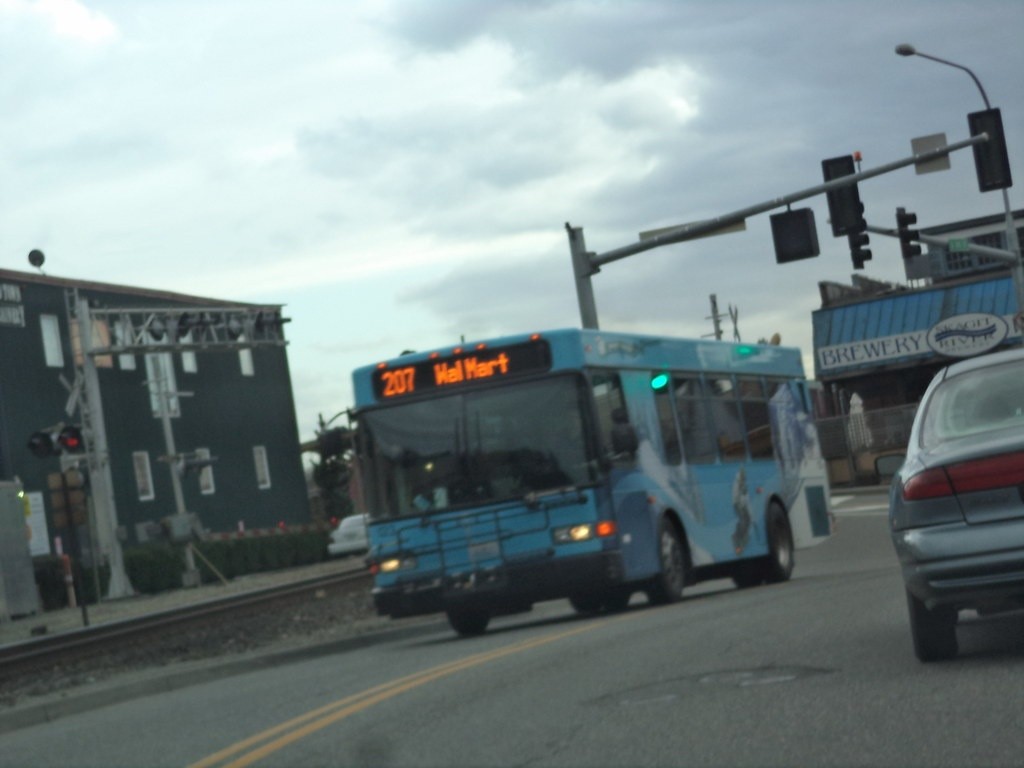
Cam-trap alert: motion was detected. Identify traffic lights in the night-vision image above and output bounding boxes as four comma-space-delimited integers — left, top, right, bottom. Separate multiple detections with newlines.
648, 374, 670, 395
249, 311, 282, 339
897, 209, 921, 258
25, 431, 53, 459
168, 312, 193, 339
58, 427, 83, 454
848, 219, 872, 269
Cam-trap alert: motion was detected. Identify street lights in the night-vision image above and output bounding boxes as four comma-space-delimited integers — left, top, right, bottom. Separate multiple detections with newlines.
896, 43, 1024, 310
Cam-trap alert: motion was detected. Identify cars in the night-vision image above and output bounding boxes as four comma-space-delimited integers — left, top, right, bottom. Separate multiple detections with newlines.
328, 512, 370, 556
874, 347, 1024, 663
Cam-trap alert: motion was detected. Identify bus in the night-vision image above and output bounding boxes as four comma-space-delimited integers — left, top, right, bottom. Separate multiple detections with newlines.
346, 327, 835, 637
